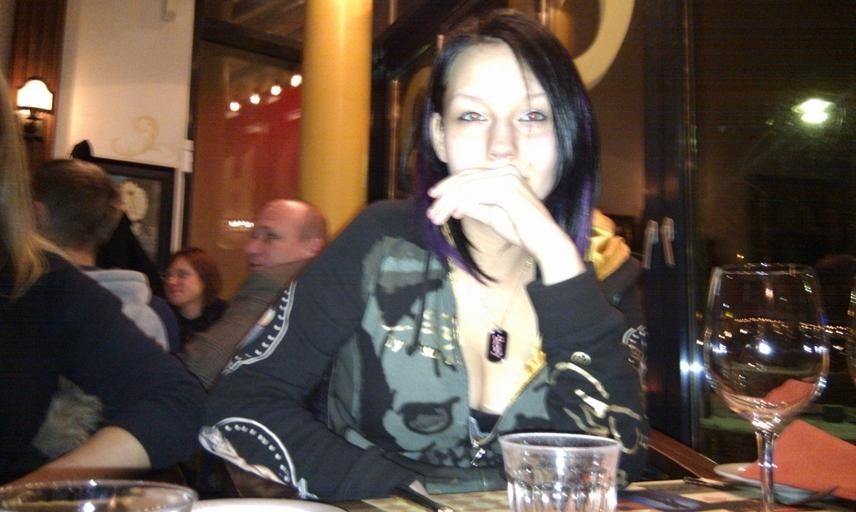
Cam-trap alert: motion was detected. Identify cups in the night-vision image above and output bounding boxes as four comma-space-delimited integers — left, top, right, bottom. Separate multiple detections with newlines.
0, 479, 197, 512
496, 429, 619, 511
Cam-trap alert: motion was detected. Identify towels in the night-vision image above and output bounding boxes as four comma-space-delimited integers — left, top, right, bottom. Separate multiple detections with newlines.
736, 421, 856, 501
763, 375, 818, 405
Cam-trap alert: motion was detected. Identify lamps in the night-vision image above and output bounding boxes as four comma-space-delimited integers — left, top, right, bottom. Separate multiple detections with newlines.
15, 76, 55, 143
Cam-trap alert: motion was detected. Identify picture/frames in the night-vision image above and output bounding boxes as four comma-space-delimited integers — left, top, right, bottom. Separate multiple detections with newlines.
89, 156, 175, 285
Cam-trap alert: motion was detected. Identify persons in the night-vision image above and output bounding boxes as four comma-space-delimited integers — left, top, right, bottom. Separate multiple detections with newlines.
170, 194, 330, 390
25, 156, 233, 355
197, 8, 648, 507
589, 208, 646, 327
0, 71, 225, 505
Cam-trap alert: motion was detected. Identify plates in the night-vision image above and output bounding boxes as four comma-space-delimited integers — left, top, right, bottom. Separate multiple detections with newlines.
183, 498, 344, 512
711, 461, 814, 503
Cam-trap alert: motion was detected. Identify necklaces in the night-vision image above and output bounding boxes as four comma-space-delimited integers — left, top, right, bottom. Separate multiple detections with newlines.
457, 261, 533, 360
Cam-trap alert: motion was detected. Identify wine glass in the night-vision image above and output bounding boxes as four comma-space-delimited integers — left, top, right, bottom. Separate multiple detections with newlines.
700, 261, 830, 511
